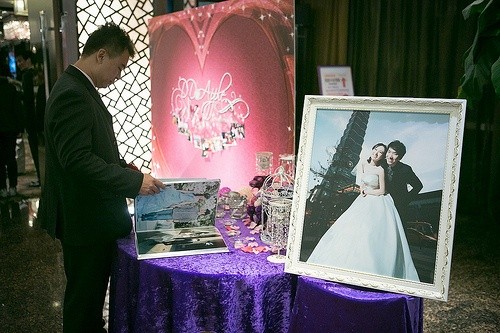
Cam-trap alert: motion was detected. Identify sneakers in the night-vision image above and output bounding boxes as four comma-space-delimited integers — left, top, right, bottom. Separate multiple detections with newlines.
9, 187, 16, 196
0, 189, 7, 197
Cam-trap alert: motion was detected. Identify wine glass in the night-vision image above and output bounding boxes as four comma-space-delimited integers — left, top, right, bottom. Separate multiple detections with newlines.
266, 199, 292, 263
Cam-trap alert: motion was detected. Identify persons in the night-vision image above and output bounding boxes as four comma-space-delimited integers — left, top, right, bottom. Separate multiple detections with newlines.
0, 48, 41, 196
38, 21, 163, 333
350, 139, 424, 282
136, 186, 210, 222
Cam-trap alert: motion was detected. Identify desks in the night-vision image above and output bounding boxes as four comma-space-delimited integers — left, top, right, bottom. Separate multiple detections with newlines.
108, 205, 424, 333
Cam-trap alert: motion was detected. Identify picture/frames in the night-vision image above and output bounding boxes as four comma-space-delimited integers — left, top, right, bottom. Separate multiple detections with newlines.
283, 95, 467, 303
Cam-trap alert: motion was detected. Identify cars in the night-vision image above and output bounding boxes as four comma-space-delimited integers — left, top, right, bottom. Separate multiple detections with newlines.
407, 227, 437, 247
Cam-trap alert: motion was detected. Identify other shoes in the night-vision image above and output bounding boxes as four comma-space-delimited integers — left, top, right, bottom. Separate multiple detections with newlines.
29, 179, 40, 186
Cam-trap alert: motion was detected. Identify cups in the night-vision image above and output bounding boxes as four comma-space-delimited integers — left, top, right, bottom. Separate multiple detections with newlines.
255, 152, 274, 176
